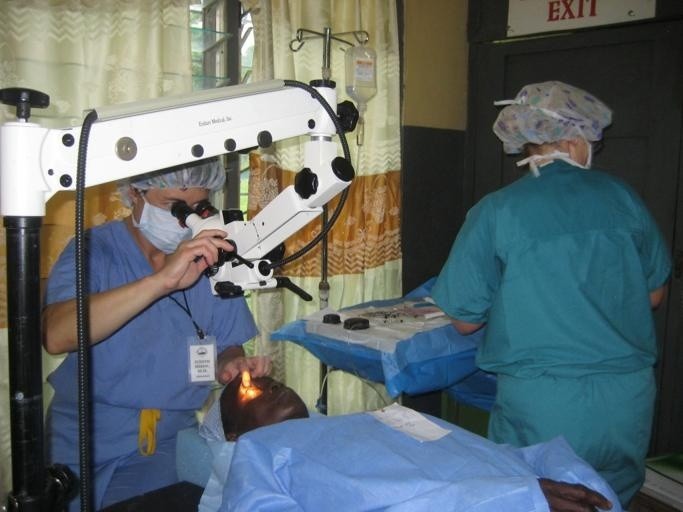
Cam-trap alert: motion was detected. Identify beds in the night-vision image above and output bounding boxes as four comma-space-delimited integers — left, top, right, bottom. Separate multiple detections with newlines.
96, 480, 204, 511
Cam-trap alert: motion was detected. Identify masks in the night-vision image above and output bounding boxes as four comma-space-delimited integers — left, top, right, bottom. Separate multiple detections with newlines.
131, 189, 192, 254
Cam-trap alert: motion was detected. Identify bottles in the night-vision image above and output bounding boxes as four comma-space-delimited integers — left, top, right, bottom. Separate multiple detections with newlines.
345, 46, 377, 111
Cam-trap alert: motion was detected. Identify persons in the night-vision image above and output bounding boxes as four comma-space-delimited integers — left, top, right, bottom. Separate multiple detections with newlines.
222, 371, 625, 512
431, 79, 674, 511
45, 148, 275, 512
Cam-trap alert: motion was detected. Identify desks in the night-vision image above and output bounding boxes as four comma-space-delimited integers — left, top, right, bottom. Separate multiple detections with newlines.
269, 295, 479, 399
